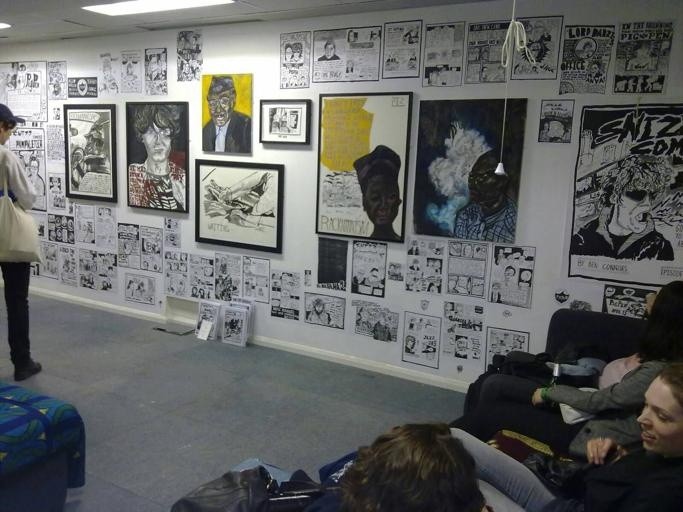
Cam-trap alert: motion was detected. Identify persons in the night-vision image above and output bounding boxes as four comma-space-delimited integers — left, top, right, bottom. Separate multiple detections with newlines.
0, 102, 42, 382
299, 273, 682, 511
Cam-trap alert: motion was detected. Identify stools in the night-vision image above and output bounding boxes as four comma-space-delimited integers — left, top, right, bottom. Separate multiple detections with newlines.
0, 386, 89, 509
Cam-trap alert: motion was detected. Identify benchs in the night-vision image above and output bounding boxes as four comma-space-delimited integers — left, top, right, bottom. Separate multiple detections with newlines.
445, 305, 649, 438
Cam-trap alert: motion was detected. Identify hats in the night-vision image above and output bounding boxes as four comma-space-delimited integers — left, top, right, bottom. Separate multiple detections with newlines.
207, 75, 237, 96
351, 143, 403, 195
0, 103, 26, 124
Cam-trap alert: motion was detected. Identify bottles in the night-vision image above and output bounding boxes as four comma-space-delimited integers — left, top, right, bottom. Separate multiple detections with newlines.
549, 362, 567, 411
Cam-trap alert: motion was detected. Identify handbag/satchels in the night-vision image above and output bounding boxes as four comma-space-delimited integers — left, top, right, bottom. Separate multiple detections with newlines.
0, 195, 42, 264
523, 452, 600, 498
558, 385, 602, 425
167, 463, 322, 511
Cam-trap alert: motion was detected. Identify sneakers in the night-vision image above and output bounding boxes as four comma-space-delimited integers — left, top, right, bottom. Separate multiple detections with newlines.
14, 359, 44, 383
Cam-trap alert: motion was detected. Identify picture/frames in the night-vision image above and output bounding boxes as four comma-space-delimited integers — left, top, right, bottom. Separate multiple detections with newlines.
194, 159, 285, 255
194, 294, 255, 347
259, 98, 312, 144
314, 90, 414, 244
63, 103, 117, 204
125, 102, 189, 214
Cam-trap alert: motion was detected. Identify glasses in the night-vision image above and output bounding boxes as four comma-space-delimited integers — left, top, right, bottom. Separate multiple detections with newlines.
621, 188, 660, 203
